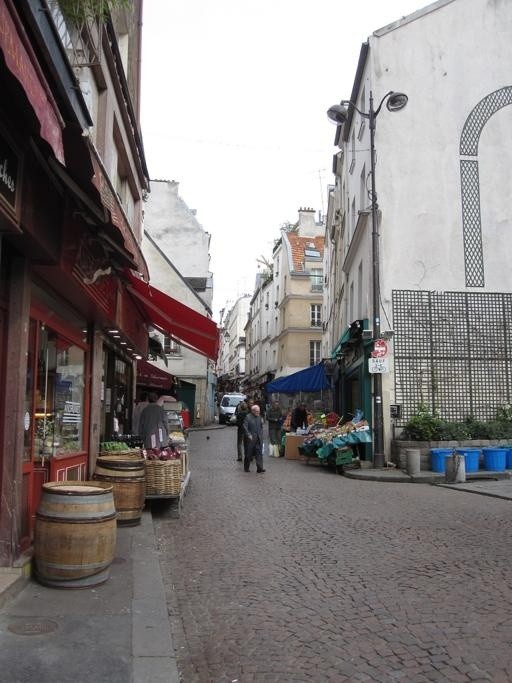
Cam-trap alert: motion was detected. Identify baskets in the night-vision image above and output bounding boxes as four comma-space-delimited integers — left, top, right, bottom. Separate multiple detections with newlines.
146, 459, 182, 495
100, 450, 141, 456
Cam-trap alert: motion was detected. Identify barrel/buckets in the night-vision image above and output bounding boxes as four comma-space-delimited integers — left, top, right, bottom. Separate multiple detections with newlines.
34, 480, 116, 588
454, 448, 481, 473
507, 447, 512, 469
91, 455, 148, 527
430, 448, 452, 472
483, 448, 508, 471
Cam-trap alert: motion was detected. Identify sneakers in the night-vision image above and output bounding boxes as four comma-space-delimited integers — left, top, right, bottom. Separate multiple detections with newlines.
237, 458, 265, 473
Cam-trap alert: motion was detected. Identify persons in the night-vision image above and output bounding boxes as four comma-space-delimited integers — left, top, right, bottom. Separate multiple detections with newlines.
235, 398, 308, 473
132, 391, 170, 449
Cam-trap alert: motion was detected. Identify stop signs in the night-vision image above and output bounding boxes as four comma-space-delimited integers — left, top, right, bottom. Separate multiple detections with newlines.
371, 339, 388, 357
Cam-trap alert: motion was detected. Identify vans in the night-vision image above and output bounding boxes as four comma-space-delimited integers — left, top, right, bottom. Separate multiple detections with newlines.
218, 392, 247, 423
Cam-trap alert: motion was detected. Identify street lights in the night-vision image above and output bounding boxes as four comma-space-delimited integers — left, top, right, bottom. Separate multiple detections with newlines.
328, 89, 410, 462
